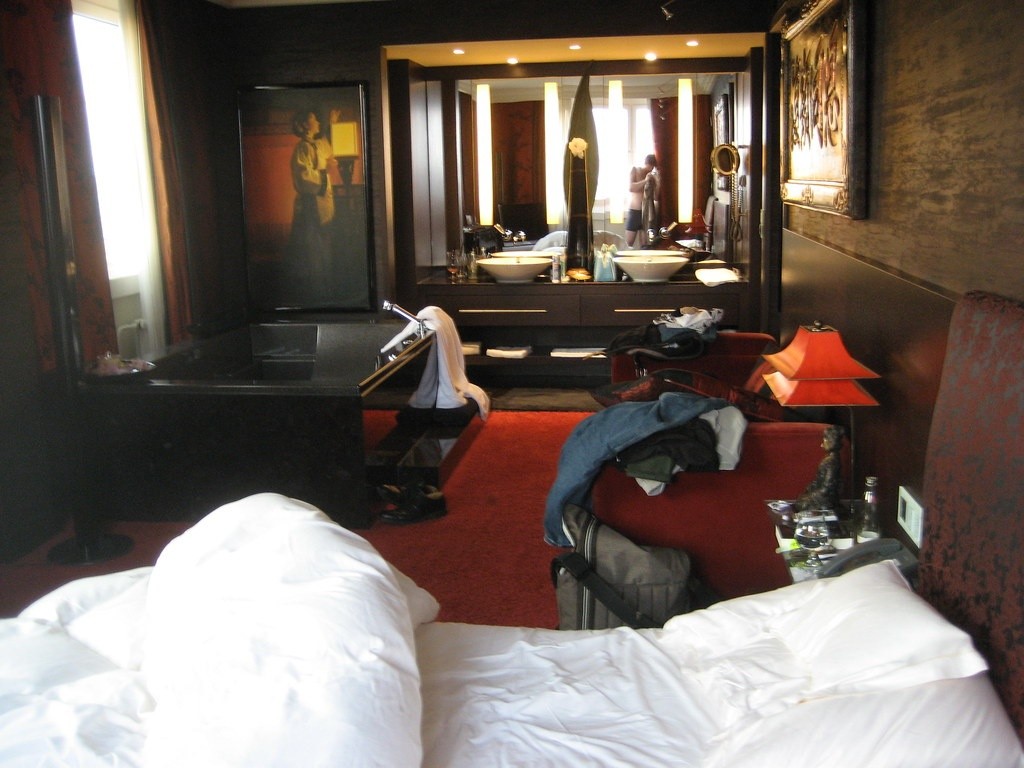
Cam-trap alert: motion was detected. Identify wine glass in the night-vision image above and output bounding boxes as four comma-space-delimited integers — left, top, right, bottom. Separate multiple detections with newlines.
793, 510, 829, 571
446, 251, 459, 280
456, 253, 466, 278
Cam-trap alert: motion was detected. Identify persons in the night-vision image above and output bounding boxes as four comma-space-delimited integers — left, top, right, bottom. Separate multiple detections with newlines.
625, 155, 659, 246
795, 425, 844, 511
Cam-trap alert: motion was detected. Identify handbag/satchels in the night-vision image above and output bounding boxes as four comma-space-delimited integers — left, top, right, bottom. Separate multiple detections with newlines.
553, 503, 694, 628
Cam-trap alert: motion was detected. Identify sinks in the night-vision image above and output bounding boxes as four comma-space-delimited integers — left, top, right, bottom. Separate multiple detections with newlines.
612, 256, 690, 283
615, 250, 685, 256
489, 250, 561, 258
476, 257, 553, 284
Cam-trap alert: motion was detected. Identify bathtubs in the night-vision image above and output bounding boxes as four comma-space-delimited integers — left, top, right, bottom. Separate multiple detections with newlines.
142, 319, 421, 389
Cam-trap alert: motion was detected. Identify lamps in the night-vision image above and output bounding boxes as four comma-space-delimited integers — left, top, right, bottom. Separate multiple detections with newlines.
759, 321, 882, 552
684, 207, 713, 249
660, 0, 675, 20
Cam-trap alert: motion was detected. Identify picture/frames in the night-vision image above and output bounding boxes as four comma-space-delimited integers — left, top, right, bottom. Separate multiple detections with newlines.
780, 0, 869, 223
235, 78, 378, 318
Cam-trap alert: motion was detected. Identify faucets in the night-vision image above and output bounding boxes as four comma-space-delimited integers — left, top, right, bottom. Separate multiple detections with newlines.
381, 299, 429, 341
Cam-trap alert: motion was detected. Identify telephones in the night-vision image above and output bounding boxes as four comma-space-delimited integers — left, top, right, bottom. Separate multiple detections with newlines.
818, 537, 919, 588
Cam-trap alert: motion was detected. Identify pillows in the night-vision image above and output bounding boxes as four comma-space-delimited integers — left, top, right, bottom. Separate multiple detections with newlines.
660, 557, 990, 744
675, 238, 706, 249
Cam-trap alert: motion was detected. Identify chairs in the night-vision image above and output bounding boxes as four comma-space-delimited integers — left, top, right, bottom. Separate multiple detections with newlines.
558, 329, 852, 606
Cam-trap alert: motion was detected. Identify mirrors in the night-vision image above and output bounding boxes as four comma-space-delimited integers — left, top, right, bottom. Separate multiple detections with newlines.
388, 31, 768, 290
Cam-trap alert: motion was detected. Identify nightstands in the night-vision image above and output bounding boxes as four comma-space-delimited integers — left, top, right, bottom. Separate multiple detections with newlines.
761, 496, 921, 586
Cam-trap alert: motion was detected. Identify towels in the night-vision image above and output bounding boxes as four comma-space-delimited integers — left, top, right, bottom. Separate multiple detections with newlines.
378, 306, 492, 422
405, 338, 437, 408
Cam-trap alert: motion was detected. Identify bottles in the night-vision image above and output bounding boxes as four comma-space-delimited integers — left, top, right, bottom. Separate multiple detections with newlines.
551, 254, 560, 283
856, 476, 882, 544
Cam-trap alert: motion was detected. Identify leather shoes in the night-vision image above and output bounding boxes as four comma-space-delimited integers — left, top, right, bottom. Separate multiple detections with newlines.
378, 483, 437, 505
380, 492, 447, 524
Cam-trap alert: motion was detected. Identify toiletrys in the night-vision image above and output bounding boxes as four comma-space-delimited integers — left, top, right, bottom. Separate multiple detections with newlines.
97, 349, 145, 373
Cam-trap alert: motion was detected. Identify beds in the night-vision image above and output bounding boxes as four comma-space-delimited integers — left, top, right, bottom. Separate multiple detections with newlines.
0, 288, 1024, 768
530, 200, 731, 260
